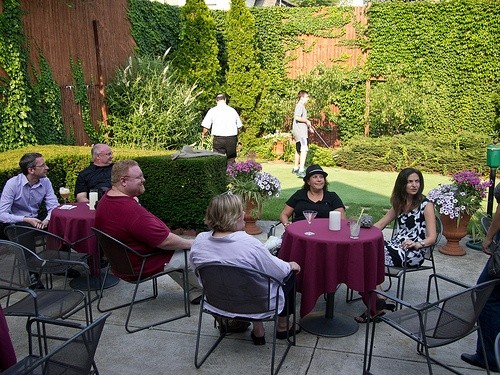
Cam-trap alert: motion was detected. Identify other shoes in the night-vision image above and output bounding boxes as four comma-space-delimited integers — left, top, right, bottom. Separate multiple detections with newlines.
292, 168, 299, 174
460, 352, 487, 367
54, 268, 79, 278
28, 274, 45, 289
297, 171, 305, 177
192, 295, 203, 304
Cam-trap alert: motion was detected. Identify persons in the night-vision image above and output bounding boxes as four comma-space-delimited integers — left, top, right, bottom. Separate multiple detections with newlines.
201, 94, 243, 161
74, 143, 139, 203
279, 164, 345, 227
0, 153, 79, 290
460, 182, 500, 373
189, 194, 301, 346
95, 159, 203, 304
354, 167, 437, 323
293, 90, 313, 178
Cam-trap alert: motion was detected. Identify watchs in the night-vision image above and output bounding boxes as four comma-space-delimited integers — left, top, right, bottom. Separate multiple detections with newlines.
420, 241, 425, 246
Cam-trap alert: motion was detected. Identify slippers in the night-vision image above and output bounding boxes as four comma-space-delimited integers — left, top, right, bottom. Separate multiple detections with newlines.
355, 311, 385, 323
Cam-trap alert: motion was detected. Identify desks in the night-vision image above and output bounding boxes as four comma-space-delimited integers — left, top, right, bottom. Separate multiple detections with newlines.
45, 201, 119, 291
277, 220, 385, 339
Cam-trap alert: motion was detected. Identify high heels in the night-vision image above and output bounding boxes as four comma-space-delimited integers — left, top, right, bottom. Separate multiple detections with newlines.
276, 323, 300, 339
251, 328, 266, 345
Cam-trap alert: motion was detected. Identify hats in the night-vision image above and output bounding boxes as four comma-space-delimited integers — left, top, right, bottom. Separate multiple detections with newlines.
303, 165, 328, 181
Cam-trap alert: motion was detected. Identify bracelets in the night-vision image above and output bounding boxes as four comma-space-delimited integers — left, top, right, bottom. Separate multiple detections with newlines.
284, 222, 291, 225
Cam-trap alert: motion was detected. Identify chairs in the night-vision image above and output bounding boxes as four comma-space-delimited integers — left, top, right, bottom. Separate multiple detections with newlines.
363, 274, 500, 375
0, 211, 296, 375
344, 210, 440, 322
480, 217, 496, 249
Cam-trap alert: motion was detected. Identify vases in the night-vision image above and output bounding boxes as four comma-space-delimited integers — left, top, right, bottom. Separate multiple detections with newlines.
438, 211, 471, 257
240, 197, 264, 235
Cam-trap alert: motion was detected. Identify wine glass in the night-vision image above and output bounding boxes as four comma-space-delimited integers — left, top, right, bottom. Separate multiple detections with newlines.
303, 210, 318, 236
59, 186, 70, 205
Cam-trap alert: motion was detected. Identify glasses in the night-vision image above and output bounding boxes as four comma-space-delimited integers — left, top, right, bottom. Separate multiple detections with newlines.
32, 163, 46, 169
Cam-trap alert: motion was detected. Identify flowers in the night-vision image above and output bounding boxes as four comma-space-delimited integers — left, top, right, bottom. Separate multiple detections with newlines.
426, 169, 494, 229
226, 160, 281, 217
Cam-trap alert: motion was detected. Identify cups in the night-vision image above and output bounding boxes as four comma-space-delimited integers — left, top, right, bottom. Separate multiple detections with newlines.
90, 190, 98, 192
350, 216, 361, 239
